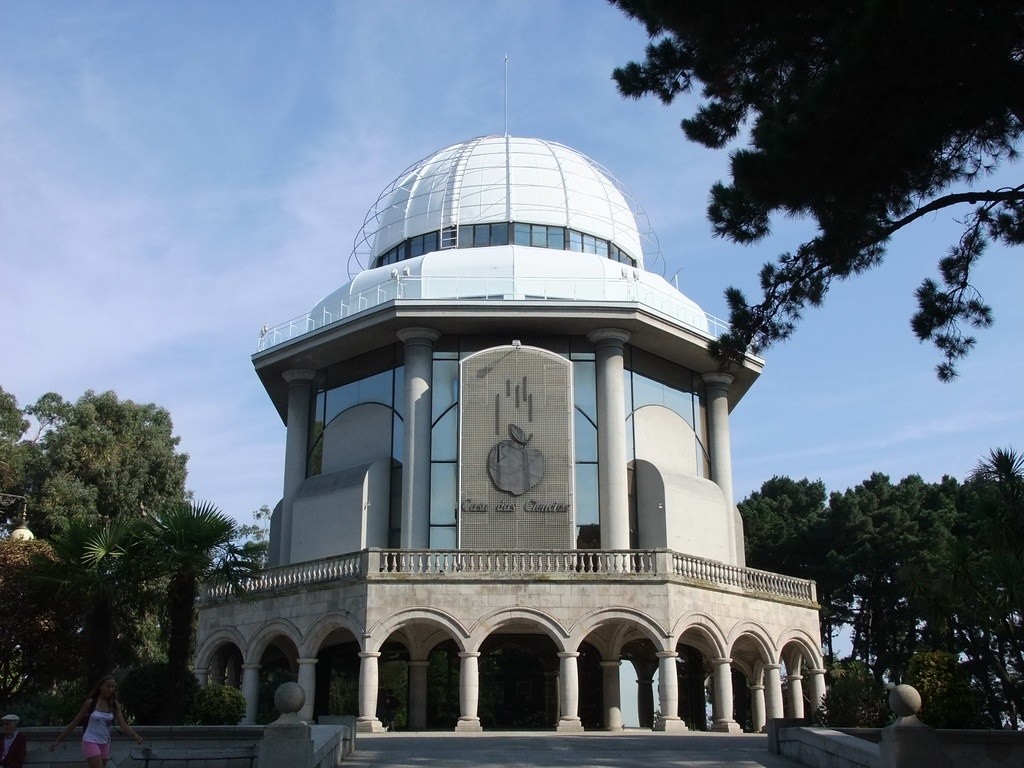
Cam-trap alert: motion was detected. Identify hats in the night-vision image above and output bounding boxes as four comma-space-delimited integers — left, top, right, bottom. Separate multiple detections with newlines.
1, 714, 20, 721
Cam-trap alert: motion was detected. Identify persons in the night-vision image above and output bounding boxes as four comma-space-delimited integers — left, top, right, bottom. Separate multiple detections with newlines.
814, 709, 824, 724
48, 675, 144, 768
587, 704, 601, 722
446, 698, 456, 727
484, 698, 514, 726
383, 688, 400, 731
0, 714, 27, 768
